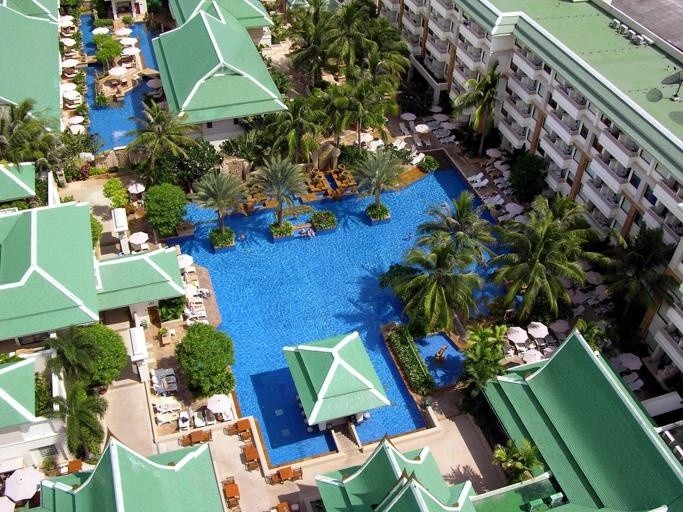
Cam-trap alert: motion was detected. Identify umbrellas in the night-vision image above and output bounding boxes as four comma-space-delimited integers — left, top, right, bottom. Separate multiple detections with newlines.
495, 261, 645, 373
205, 393, 233, 413
184, 282, 195, 300
176, 252, 194, 270
4, 466, 40, 502
60, 13, 161, 195
397, 104, 527, 225
127, 230, 149, 245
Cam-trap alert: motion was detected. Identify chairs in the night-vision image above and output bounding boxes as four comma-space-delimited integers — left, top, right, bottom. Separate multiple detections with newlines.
179, 254, 208, 327
397, 102, 530, 223
507, 254, 646, 393
147, 366, 303, 511
